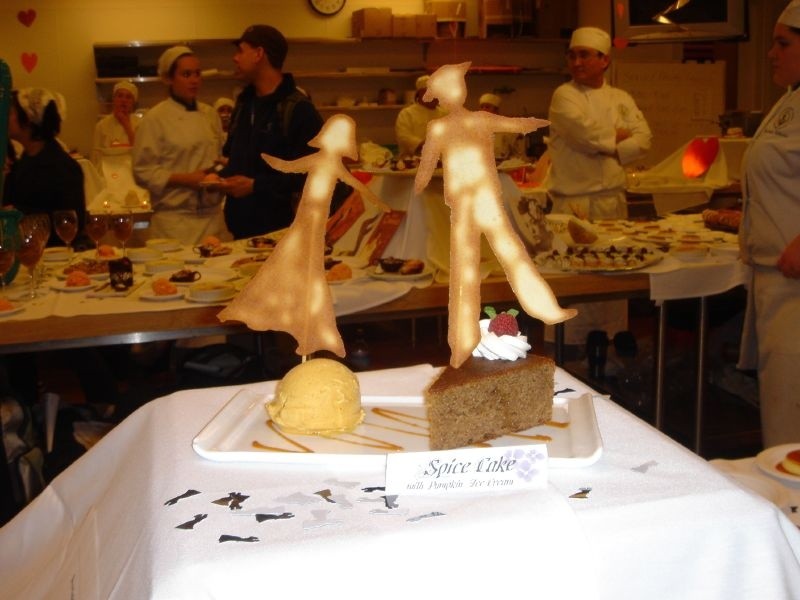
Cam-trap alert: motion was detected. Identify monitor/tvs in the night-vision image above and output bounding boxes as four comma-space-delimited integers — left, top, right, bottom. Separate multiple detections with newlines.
612, 0, 748, 41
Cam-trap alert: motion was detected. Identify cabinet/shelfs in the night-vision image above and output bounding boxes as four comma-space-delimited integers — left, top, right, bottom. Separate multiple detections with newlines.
93, 37, 559, 124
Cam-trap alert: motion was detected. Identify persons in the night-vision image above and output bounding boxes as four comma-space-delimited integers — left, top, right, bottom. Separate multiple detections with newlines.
394, 74, 524, 168
537, 26, 652, 221
0, 22, 328, 417
737, 0, 799, 450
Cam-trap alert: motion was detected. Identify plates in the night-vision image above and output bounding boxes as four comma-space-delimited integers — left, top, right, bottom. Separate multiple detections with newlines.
46, 237, 435, 304
0, 301, 26, 315
199, 181, 226, 184
94, 147, 133, 154
755, 442, 800, 485
191, 391, 605, 469
535, 214, 738, 274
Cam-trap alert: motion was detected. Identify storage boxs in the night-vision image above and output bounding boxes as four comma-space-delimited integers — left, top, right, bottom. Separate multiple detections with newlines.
415, 15, 437, 36
352, 8, 391, 38
393, 14, 416, 36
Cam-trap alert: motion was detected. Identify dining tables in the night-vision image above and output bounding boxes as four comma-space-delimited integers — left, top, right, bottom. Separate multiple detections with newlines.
0, 217, 750, 520
0, 365, 800, 600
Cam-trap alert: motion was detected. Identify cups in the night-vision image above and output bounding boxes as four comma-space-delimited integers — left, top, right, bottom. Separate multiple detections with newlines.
404, 90, 416, 103
545, 213, 573, 234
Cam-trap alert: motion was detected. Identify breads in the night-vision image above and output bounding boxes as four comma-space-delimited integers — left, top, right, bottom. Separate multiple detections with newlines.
199, 235, 224, 257
0, 299, 12, 312
151, 278, 178, 295
202, 173, 220, 182
568, 220, 598, 244
64, 271, 91, 286
99, 244, 116, 257
702, 207, 742, 227
325, 263, 352, 281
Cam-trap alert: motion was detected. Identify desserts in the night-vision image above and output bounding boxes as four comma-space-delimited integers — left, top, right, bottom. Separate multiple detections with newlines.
251, 237, 276, 248
169, 270, 196, 282
377, 256, 424, 274
216, 111, 392, 435
411, 63, 577, 451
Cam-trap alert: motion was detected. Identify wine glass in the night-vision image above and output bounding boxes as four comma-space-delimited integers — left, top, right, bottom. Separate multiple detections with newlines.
0, 210, 134, 301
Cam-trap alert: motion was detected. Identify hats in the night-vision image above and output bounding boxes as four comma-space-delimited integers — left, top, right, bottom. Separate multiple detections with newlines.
158, 45, 193, 73
570, 27, 611, 55
480, 94, 501, 107
113, 82, 139, 102
215, 98, 236, 111
236, 25, 288, 59
778, 0, 800, 28
416, 75, 430, 89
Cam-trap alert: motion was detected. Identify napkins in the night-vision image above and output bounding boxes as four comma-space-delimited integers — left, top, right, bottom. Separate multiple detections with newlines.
357, 364, 435, 404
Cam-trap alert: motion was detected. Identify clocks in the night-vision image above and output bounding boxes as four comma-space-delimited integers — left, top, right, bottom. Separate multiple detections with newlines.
309, 0, 346, 16
